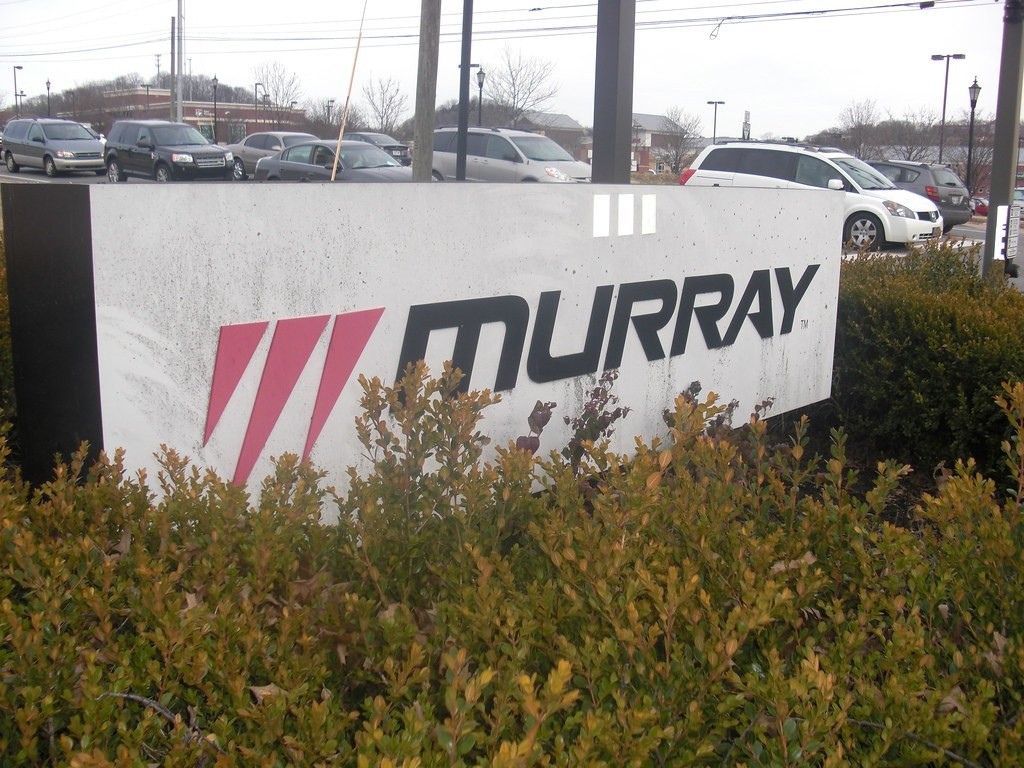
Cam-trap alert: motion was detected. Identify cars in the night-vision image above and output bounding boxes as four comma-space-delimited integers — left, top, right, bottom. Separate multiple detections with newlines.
332, 130, 413, 167
969, 196, 991, 218
251, 139, 436, 184
223, 132, 323, 182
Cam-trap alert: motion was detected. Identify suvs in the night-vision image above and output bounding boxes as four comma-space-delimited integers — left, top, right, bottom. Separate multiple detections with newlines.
860, 157, 973, 236
101, 120, 237, 184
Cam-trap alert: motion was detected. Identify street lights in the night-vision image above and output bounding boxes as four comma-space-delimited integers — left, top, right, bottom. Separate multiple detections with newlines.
14, 65, 24, 121
212, 74, 220, 145
45, 79, 52, 118
476, 67, 487, 127
931, 52, 966, 166
966, 76, 982, 189
708, 100, 725, 144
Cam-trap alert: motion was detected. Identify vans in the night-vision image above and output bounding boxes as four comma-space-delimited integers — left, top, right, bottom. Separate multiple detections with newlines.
1011, 185, 1024, 220
429, 124, 595, 184
677, 139, 944, 252
0, 117, 110, 178
